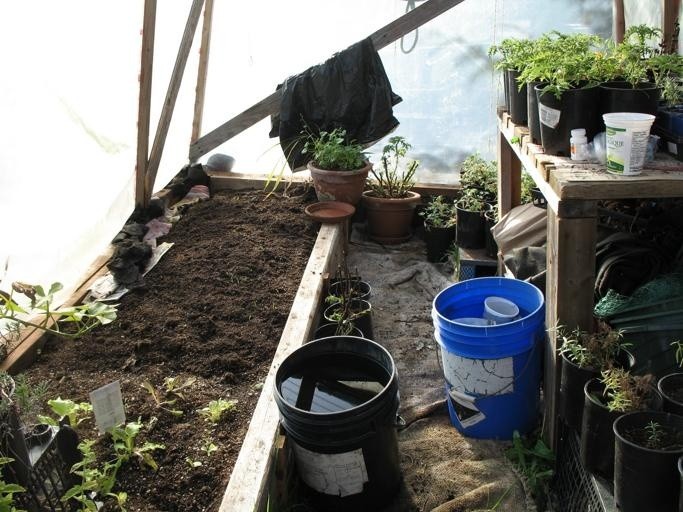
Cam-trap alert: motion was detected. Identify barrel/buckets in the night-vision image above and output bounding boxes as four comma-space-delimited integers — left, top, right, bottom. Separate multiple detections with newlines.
431, 276, 544, 448
599, 112, 656, 178
271, 335, 400, 511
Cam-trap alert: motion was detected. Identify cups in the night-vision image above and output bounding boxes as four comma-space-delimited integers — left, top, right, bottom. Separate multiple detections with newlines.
483, 295, 519, 323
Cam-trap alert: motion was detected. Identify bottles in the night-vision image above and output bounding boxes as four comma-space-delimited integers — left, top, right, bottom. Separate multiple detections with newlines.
570, 128, 588, 161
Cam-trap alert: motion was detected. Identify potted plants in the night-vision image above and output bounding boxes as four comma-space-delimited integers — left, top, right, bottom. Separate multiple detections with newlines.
547, 318, 683, 512
268, 113, 422, 244
311, 254, 372, 339
489, 24, 683, 157
421, 156, 539, 261
